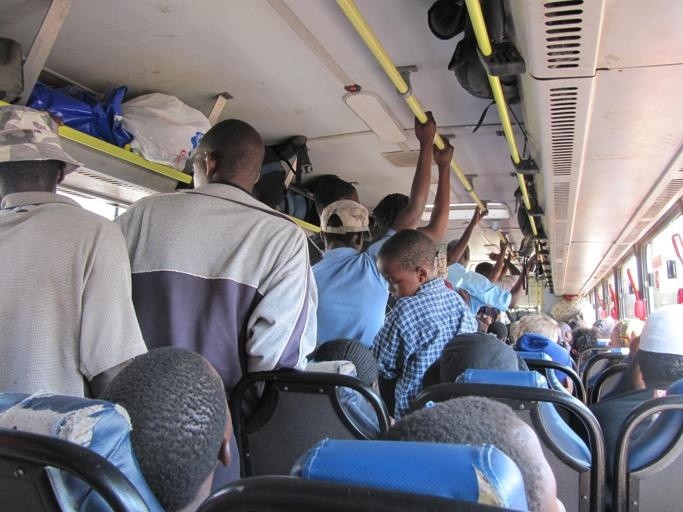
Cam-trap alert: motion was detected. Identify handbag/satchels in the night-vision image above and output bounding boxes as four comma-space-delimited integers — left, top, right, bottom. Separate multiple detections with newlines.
122, 92, 212, 171
253, 135, 317, 223
427, 0, 544, 296
28, 82, 134, 148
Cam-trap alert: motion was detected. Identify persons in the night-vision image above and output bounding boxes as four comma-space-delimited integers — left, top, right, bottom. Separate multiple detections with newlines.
100, 346, 233, 511
1, 104, 150, 398
305, 295, 681, 511
307, 110, 536, 350
111, 119, 319, 418
369, 229, 477, 422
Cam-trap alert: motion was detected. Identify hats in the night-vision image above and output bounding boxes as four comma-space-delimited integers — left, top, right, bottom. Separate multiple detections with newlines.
639, 305, 683, 355
514, 333, 572, 381
0, 105, 81, 175
321, 198, 373, 242
423, 332, 530, 388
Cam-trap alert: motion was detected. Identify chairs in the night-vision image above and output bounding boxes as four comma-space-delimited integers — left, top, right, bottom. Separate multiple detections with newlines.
1, 331, 682, 512
